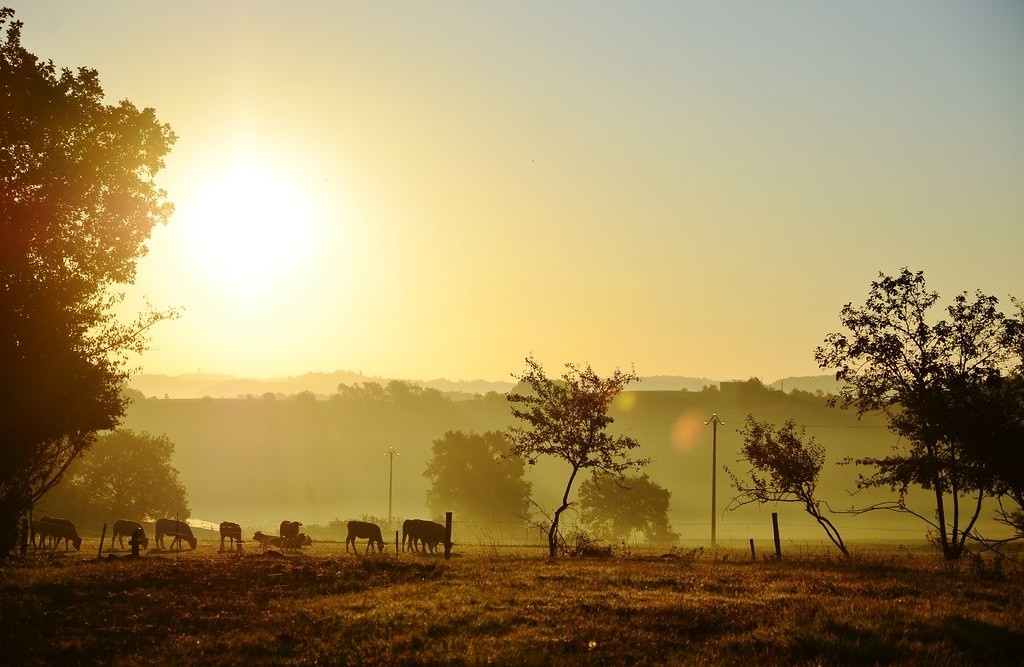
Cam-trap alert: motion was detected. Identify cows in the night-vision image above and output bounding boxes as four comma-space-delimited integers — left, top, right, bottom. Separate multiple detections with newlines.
29, 515, 83, 551
155, 518, 197, 550
219, 521, 246, 552
112, 520, 149, 551
346, 521, 388, 554
252, 521, 312, 552
401, 519, 456, 555
